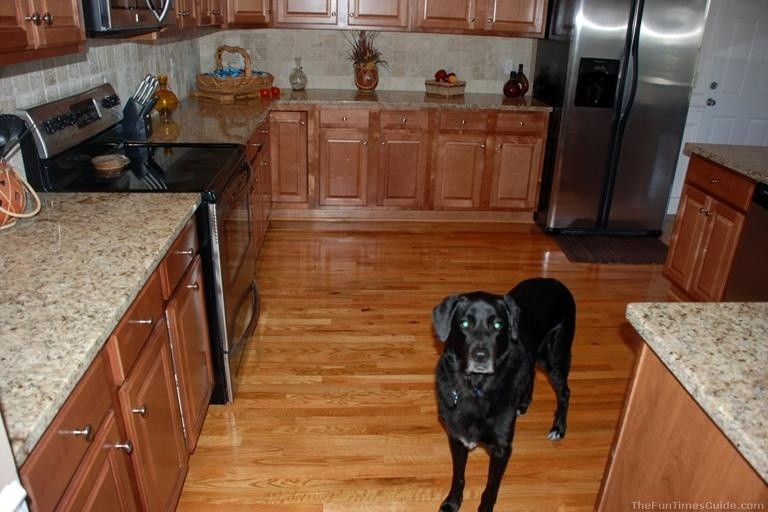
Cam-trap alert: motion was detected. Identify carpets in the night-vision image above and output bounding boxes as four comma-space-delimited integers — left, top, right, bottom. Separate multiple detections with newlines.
550, 234, 670, 265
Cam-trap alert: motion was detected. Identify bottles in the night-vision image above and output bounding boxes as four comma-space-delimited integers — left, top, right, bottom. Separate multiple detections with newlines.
503, 64, 529, 97
287, 58, 308, 91
155, 116, 179, 156
290, 91, 307, 102
153, 76, 177, 116
503, 98, 526, 106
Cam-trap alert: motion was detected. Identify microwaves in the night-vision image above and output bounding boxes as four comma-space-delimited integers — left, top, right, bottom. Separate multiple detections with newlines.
83, 0, 177, 36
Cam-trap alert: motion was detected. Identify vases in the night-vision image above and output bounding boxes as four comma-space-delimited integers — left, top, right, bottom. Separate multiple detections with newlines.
288, 57, 306, 90
517, 64, 528, 97
504, 71, 521, 98
152, 74, 178, 122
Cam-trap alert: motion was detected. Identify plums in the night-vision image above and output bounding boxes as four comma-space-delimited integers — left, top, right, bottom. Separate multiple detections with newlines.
434, 70, 457, 83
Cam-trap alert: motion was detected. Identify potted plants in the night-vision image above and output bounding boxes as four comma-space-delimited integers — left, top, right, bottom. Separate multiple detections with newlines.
342, 30, 390, 93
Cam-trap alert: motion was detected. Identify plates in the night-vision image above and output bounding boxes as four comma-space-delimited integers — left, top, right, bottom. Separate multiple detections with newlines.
91, 153, 131, 169
95, 170, 129, 177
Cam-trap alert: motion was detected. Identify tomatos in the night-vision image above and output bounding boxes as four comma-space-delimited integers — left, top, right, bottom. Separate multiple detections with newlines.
271, 87, 280, 95
259, 88, 269, 96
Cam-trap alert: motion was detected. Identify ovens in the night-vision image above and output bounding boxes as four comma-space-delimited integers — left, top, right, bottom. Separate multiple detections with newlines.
208, 159, 262, 406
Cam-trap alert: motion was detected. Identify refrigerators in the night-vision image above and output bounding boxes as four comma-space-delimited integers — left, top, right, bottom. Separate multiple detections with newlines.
532, 0, 711, 238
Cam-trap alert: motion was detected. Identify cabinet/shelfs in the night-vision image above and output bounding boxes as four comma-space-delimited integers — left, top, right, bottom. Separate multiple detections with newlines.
665, 0, 768, 216
270, 107, 549, 212
157, 215, 215, 454
661, 155, 768, 302
252, 112, 270, 263
19, 269, 190, 512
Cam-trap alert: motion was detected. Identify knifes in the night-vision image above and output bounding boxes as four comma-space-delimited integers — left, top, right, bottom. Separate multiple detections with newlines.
139, 172, 172, 192
132, 73, 159, 104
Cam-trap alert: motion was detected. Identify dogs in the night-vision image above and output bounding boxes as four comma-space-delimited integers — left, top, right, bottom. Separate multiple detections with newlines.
432, 276, 577, 512
